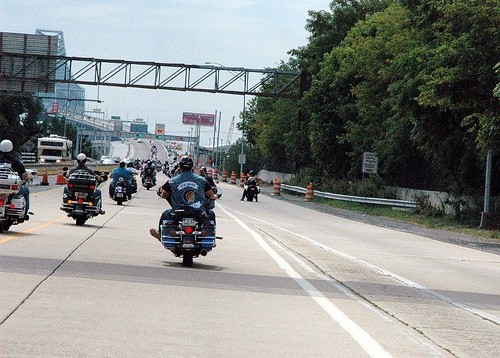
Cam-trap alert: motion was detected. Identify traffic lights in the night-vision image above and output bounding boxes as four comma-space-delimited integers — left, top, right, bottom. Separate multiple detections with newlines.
136, 133, 142, 138
157, 135, 164, 139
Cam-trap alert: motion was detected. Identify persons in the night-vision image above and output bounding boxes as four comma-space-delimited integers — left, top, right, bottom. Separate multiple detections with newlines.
0, 140, 30, 221
109, 161, 132, 200
149, 155, 218, 248
64, 153, 105, 215
132, 158, 141, 170
141, 156, 183, 191
125, 162, 139, 193
198, 167, 217, 190
240, 169, 259, 203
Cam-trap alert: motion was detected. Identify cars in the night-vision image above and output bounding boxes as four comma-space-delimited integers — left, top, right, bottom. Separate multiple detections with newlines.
74, 153, 121, 167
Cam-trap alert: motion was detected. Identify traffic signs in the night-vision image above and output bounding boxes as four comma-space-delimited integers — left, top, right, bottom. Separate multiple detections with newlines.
130, 124, 148, 133
362, 152, 377, 175
182, 112, 214, 126
114, 120, 123, 132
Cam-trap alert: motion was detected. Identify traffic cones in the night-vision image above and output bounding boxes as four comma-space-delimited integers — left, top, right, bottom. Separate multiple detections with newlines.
40, 167, 49, 186
56, 165, 66, 185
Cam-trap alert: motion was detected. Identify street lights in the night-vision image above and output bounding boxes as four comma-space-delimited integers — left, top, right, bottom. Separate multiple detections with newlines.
205, 60, 247, 174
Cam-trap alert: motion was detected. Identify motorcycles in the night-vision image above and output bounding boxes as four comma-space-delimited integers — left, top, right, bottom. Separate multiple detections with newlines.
158, 186, 221, 267
130, 172, 139, 193
112, 173, 132, 205
0, 161, 38, 234
60, 166, 109, 226
241, 179, 259, 202
204, 180, 219, 210
142, 171, 157, 190
127, 158, 195, 179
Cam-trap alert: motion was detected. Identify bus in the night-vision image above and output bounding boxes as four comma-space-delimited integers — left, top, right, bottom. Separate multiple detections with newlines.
37, 134, 73, 162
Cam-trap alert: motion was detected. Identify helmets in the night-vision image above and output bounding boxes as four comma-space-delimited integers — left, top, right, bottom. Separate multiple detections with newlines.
250, 170, 256, 176
180, 156, 194, 171
119, 161, 125, 167
147, 161, 152, 166
0, 140, 13, 152
77, 153, 87, 163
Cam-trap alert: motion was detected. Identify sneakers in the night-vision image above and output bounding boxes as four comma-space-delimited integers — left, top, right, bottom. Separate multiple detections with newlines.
150, 229, 160, 240
23, 215, 29, 220
99, 210, 105, 214
